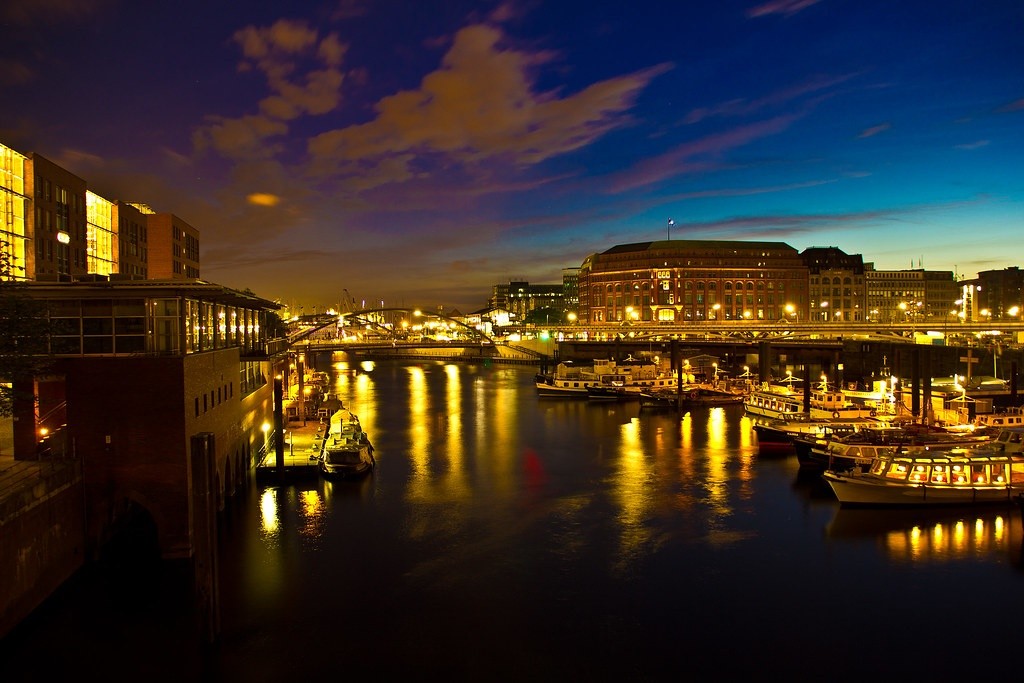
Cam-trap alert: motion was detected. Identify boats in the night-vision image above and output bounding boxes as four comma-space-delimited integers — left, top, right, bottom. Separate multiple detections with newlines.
317, 407, 377, 481
278, 362, 346, 422
533, 347, 1024, 505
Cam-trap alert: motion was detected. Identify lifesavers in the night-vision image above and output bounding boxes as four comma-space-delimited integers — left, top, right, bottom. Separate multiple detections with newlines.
833, 411, 840, 418
